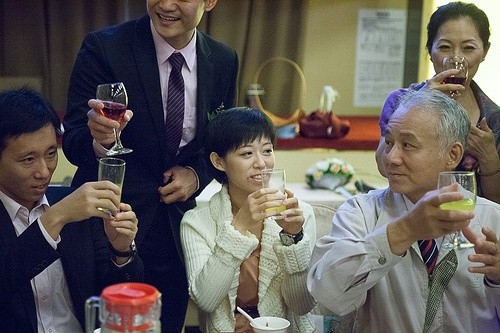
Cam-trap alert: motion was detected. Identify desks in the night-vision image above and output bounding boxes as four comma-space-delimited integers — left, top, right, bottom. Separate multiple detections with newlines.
186, 181, 347, 328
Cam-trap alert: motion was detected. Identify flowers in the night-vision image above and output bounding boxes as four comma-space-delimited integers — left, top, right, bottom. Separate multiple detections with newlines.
307, 158, 355, 198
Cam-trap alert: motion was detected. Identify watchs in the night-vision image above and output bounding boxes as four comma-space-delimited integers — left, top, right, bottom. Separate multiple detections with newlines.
110, 239, 138, 257
279, 228, 304, 246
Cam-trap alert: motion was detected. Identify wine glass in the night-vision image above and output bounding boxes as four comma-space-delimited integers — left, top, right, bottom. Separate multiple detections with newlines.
96, 82, 133, 156
437, 170, 476, 251
441, 55, 468, 99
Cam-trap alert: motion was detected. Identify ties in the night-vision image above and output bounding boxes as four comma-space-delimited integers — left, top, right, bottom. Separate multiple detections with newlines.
165, 52, 185, 149
416, 236, 440, 276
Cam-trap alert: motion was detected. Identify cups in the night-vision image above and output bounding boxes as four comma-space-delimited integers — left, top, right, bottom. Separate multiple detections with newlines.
250, 317, 291, 333
97, 158, 126, 213
262, 168, 287, 220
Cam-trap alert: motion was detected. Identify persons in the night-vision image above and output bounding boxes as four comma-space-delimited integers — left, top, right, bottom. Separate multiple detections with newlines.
180, 106, 319, 333
375, 2, 500, 204
0, 84, 143, 333
62, 0, 240, 333
307, 88, 500, 333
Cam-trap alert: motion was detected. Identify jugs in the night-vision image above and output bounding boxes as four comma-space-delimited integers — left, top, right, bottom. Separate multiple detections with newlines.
85, 282, 162, 333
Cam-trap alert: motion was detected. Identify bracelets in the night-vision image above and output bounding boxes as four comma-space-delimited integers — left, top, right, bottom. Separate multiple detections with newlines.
479, 169, 500, 177
